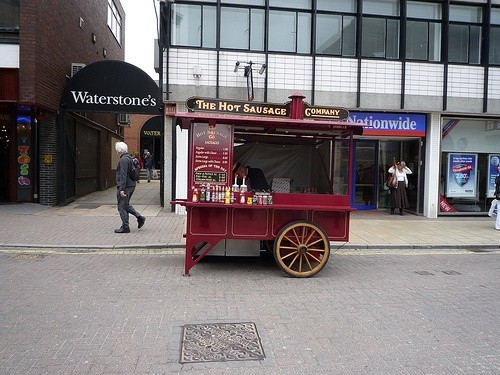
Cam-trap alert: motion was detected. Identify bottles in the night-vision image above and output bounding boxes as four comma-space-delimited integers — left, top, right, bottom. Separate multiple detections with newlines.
247, 195, 258, 205
206, 183, 210, 202
231, 191, 234, 203
212, 186, 215, 202
225, 186, 231, 204
218, 186, 222, 202
258, 196, 262, 204
200, 185, 205, 203
268, 196, 272, 204
222, 186, 225, 203
193, 189, 198, 203
239, 189, 246, 204
215, 186, 218, 202
263, 196, 267, 204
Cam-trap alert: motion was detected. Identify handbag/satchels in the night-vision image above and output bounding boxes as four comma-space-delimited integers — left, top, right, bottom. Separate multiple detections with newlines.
386, 165, 398, 189
152, 170, 158, 179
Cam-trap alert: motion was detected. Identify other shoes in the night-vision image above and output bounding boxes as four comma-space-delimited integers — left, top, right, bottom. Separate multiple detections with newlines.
399, 207, 404, 215
390, 208, 394, 215
115, 226, 130, 233
137, 216, 146, 228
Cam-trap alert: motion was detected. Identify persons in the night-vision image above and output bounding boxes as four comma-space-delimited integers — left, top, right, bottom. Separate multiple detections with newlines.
114, 142, 146, 233
388, 161, 413, 215
143, 152, 154, 182
233, 162, 268, 193
134, 153, 143, 183
495, 167, 500, 230
360, 162, 374, 205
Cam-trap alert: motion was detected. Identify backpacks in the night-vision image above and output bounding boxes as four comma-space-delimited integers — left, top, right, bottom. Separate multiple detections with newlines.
121, 156, 140, 181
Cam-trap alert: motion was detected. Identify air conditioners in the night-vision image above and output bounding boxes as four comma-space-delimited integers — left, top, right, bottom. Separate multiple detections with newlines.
120, 114, 129, 124
71, 63, 86, 78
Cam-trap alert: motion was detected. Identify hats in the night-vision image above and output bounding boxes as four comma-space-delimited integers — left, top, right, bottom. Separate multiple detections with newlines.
232, 161, 241, 174
